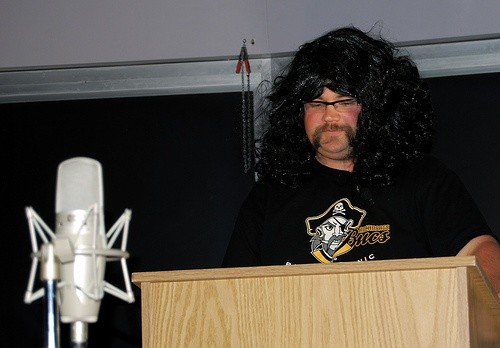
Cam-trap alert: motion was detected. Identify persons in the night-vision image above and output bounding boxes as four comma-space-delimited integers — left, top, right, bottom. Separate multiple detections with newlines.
220, 27, 500, 300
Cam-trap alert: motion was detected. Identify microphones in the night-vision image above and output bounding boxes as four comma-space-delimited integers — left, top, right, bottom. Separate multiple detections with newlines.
53, 155, 109, 324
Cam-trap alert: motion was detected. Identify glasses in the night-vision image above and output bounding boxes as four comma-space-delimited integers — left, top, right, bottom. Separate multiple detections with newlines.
304, 98, 358, 113
